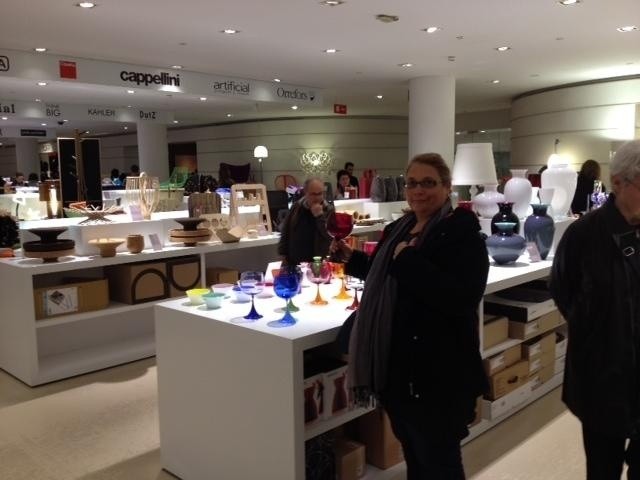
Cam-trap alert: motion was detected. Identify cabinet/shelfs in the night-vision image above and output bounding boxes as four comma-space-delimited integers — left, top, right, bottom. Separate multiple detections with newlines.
0, 181, 413, 387
102, 188, 185, 211
154, 250, 564, 480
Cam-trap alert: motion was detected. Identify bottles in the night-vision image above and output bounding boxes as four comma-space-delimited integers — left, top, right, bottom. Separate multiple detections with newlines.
312, 256, 322, 277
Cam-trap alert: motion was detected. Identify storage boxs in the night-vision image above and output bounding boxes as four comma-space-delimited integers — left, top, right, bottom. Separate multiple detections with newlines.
169, 253, 202, 298
474, 343, 532, 422
483, 294, 567, 341
358, 410, 405, 471
33, 279, 109, 320
483, 314, 509, 351
206, 267, 239, 286
104, 262, 169, 305
521, 331, 568, 392
332, 439, 366, 480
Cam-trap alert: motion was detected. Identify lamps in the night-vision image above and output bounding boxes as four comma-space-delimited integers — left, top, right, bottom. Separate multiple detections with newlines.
254, 146, 269, 184
451, 143, 499, 201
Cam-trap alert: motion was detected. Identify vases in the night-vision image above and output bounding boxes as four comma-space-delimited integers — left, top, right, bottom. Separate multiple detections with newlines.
504, 170, 532, 220
491, 202, 520, 235
527, 187, 540, 217
541, 164, 577, 220
474, 184, 505, 219
524, 204, 554, 260
485, 223, 526, 265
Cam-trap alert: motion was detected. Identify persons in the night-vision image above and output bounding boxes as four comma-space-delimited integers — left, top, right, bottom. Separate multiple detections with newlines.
344, 162, 362, 200
11, 172, 27, 188
571, 158, 608, 219
547, 137, 640, 480
27, 172, 40, 186
329, 153, 490, 480
333, 170, 355, 200
277, 178, 336, 267
109, 167, 119, 184
130, 165, 141, 177
117, 173, 128, 185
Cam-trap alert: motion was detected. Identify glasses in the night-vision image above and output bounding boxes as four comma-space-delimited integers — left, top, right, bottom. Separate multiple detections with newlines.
401, 178, 446, 190
624, 176, 639, 191
308, 191, 324, 196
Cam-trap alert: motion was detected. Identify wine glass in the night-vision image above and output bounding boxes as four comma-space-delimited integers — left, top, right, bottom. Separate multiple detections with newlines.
335, 263, 353, 301
325, 211, 353, 261
241, 271, 266, 323
344, 275, 362, 311
307, 262, 332, 307
272, 268, 303, 325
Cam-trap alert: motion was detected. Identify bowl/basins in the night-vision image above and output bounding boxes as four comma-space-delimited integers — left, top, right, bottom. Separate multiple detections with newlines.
212, 283, 235, 296
232, 285, 254, 302
186, 289, 210, 305
205, 294, 225, 310
